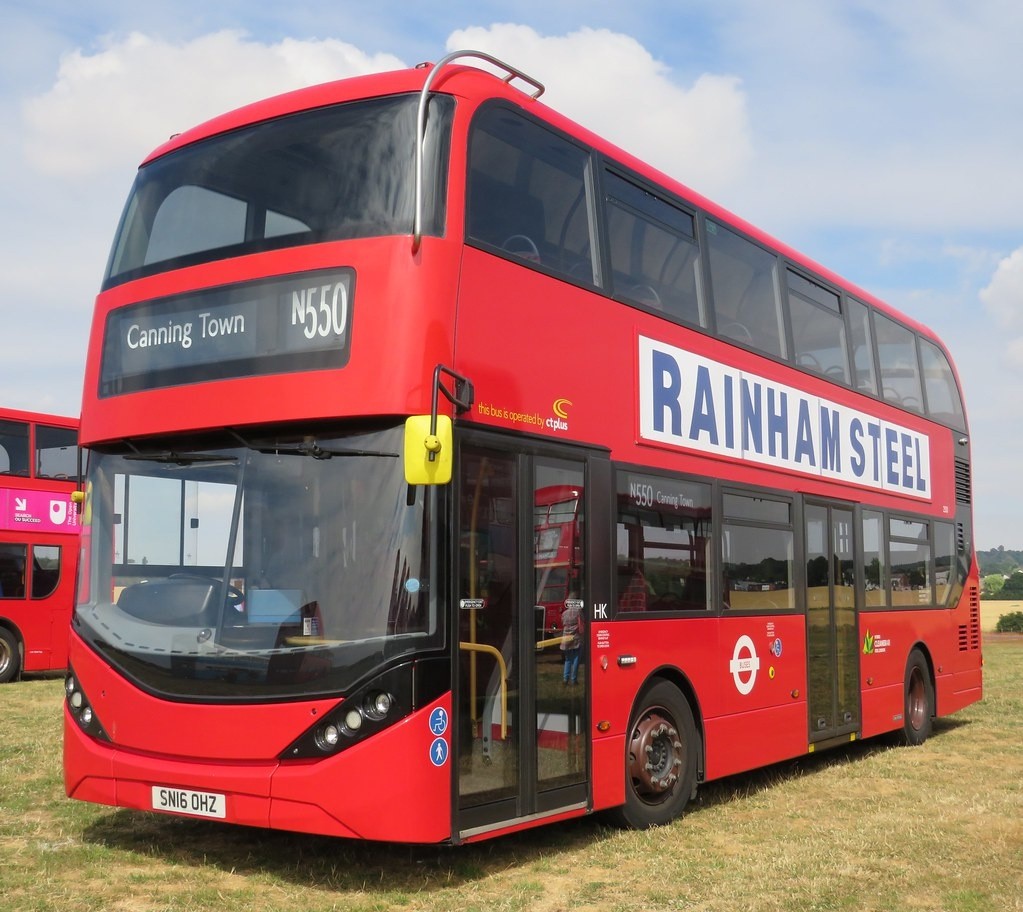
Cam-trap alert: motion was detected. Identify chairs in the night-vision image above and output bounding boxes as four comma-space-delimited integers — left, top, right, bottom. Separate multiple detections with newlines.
884, 387, 903, 406
570, 260, 594, 282
1, 470, 13, 475
16, 469, 29, 476
795, 353, 824, 374
857, 377, 872, 394
825, 365, 846, 383
903, 396, 920, 411
630, 284, 665, 311
54, 473, 68, 479
502, 234, 541, 264
722, 323, 755, 347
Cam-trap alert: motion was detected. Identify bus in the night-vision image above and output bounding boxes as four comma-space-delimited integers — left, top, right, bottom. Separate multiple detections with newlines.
65, 50, 984, 845
0, 407, 113, 683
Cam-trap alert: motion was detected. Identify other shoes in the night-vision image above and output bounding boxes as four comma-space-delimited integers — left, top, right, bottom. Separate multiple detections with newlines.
572, 681, 578, 687
562, 681, 570, 686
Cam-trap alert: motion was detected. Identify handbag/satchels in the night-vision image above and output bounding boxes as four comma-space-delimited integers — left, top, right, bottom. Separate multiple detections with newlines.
577, 612, 585, 634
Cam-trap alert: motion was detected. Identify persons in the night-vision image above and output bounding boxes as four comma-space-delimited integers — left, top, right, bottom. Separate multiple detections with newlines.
614, 524, 939, 613
559, 591, 584, 688
